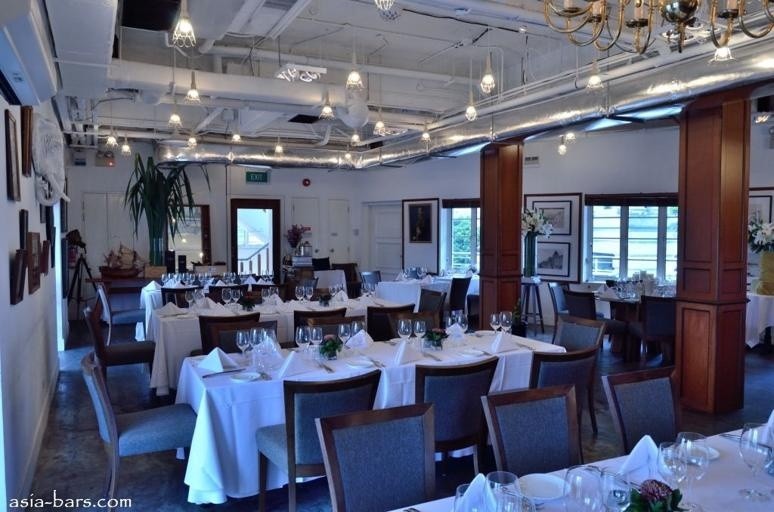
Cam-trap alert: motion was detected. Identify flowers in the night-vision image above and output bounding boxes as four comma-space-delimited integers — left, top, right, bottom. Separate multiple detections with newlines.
745, 221, 774, 251
285, 224, 303, 248
521, 211, 556, 237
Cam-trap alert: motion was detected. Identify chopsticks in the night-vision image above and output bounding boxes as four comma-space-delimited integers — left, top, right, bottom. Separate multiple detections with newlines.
201, 367, 246, 377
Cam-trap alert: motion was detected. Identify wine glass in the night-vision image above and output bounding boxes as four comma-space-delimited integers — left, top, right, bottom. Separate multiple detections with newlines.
456, 312, 468, 348
261, 289, 271, 303
294, 328, 311, 364
397, 318, 411, 354
234, 331, 251, 363
405, 264, 428, 280
155, 271, 274, 287
260, 328, 277, 363
499, 311, 512, 342
294, 286, 304, 304
413, 321, 425, 356
192, 288, 204, 315
562, 464, 601, 512
328, 284, 339, 307
445, 314, 456, 346
248, 328, 264, 362
304, 286, 315, 304
482, 471, 524, 511
309, 328, 322, 366
454, 484, 485, 512
337, 322, 349, 360
351, 319, 366, 357
360, 283, 371, 305
737, 422, 774, 497
337, 283, 346, 307
601, 463, 635, 512
231, 289, 241, 309
268, 287, 280, 303
183, 289, 195, 315
220, 288, 231, 309
653, 439, 687, 490
490, 314, 501, 342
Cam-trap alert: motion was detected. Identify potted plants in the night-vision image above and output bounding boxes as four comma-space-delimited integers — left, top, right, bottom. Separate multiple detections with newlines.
124, 153, 211, 266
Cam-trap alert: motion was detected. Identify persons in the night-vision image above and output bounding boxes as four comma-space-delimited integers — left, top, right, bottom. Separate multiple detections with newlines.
412, 206, 428, 241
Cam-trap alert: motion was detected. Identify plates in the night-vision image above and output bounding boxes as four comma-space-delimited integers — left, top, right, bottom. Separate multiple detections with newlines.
670, 431, 721, 463
466, 349, 484, 357
390, 337, 410, 346
231, 371, 262, 383
668, 444, 720, 462
343, 357, 375, 371
478, 330, 498, 337
514, 471, 571, 499
673, 429, 709, 488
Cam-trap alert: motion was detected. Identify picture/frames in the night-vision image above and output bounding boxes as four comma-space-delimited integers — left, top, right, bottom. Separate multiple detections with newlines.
402, 198, 440, 275
4, 104, 56, 306
523, 194, 583, 283
61, 175, 70, 299
748, 187, 773, 224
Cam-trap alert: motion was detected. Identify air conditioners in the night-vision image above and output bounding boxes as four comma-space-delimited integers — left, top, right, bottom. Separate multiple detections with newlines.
0, 0, 59, 108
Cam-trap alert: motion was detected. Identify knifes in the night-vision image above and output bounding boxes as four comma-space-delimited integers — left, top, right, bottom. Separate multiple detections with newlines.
515, 339, 538, 353
478, 346, 490, 357
363, 352, 384, 370
420, 348, 441, 363
259, 372, 273, 381
719, 428, 771, 450
314, 357, 333, 374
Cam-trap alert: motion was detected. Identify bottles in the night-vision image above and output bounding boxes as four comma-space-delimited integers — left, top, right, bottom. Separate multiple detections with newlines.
615, 271, 677, 298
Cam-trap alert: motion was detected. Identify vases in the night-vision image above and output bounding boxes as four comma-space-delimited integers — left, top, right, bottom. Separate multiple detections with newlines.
754, 251, 774, 295
523, 235, 537, 275
